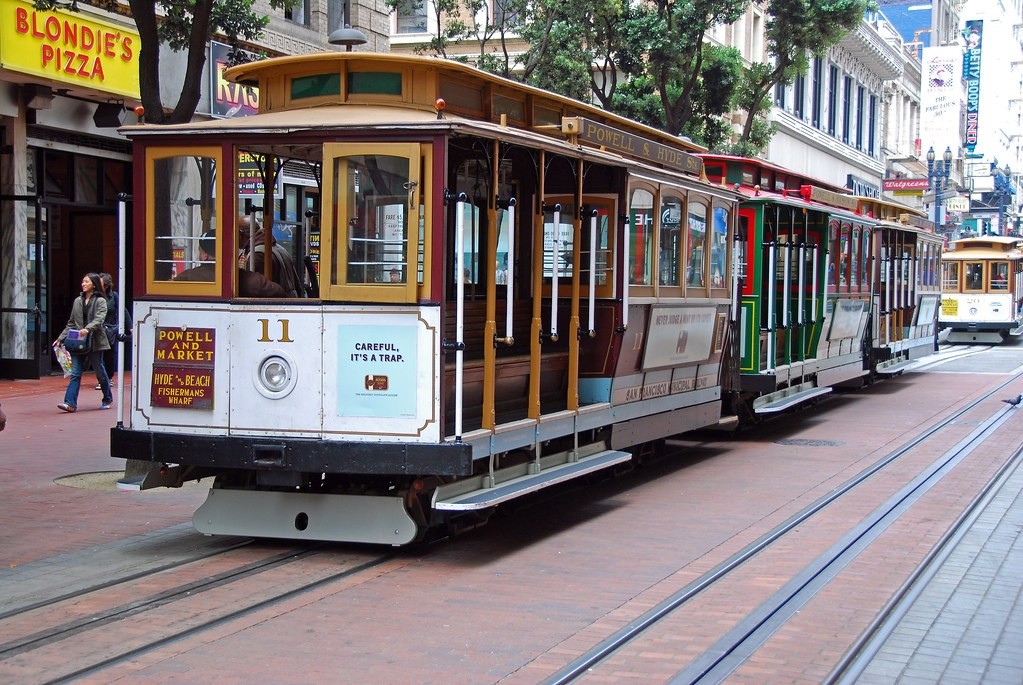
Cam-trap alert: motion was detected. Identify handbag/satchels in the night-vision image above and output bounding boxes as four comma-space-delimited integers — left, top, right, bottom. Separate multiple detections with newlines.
63, 328, 91, 355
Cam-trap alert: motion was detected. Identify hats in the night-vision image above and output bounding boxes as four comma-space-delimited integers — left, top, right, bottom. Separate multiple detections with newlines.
198, 229, 216, 255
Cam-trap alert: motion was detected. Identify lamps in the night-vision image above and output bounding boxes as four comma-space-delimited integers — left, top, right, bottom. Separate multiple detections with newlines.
93, 98, 127, 128
22, 83, 53, 110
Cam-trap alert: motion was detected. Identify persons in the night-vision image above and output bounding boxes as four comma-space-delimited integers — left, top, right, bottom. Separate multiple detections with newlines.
173, 229, 288, 299
464, 268, 472, 284
933, 70, 945, 86
963, 29, 981, 49
96, 273, 133, 389
238, 215, 304, 297
390, 268, 400, 282
51, 273, 114, 413
495, 260, 508, 284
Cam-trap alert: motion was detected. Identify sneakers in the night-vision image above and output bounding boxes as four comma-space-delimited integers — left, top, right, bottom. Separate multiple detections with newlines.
100, 402, 114, 408
57, 403, 75, 412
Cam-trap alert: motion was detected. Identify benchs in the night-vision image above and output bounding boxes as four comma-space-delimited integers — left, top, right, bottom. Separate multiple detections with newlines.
761, 300, 796, 362
443, 300, 583, 423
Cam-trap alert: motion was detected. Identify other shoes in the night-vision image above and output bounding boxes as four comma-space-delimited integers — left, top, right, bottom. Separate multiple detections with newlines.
110, 377, 114, 386
95, 383, 101, 389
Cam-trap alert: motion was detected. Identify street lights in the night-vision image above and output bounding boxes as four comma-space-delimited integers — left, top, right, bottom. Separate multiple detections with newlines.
923, 146, 953, 235
991, 164, 1012, 236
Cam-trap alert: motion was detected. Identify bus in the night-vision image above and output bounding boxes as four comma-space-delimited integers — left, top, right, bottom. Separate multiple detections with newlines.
840, 193, 948, 388
941, 234, 1023, 345
589, 152, 882, 433
108, 49, 754, 552
